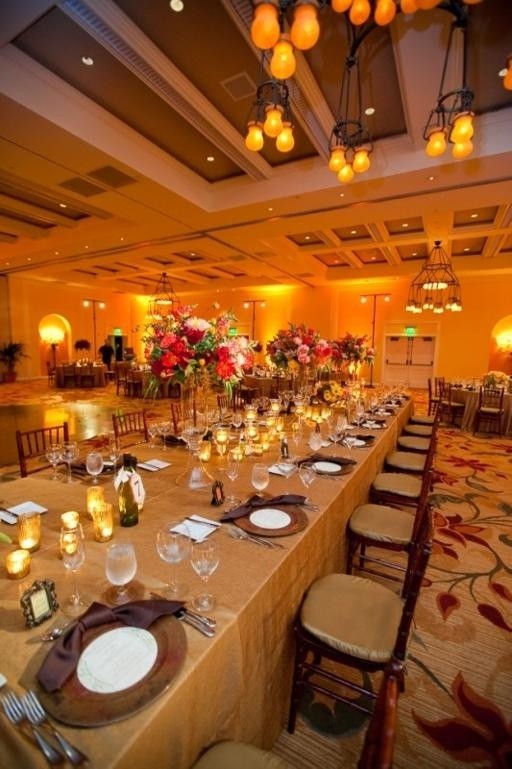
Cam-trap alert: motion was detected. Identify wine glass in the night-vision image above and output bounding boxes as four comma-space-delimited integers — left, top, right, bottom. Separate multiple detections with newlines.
327, 382, 409, 461
44, 399, 317, 615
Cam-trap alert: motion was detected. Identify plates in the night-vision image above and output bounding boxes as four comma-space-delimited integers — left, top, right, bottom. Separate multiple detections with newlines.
78, 627, 158, 694
313, 461, 341, 472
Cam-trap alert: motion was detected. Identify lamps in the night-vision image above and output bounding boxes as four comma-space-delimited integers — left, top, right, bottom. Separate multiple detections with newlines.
406, 241, 464, 313
246, 0, 512, 183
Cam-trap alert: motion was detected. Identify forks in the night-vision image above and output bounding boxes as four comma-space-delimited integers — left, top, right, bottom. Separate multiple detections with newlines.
0, 689, 88, 768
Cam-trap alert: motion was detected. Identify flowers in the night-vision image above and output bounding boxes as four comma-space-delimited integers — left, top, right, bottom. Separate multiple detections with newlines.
141, 303, 261, 397
264, 321, 333, 378
331, 333, 375, 368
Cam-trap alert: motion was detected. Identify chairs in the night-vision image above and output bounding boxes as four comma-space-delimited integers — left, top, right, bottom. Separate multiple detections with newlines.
171, 400, 196, 434
112, 409, 150, 449
233, 385, 242, 411
0, 399, 416, 769
272, 384, 282, 398
114, 361, 181, 397
218, 395, 227, 421
16, 421, 69, 476
47, 360, 108, 387
428, 377, 512, 439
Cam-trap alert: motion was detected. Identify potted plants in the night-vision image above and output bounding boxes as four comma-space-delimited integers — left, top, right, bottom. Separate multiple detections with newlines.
0, 342, 30, 382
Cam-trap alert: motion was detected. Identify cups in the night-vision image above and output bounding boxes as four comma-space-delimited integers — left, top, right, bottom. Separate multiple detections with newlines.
4, 485, 113, 580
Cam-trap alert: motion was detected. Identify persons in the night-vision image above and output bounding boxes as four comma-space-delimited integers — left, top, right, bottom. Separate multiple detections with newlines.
99, 338, 114, 381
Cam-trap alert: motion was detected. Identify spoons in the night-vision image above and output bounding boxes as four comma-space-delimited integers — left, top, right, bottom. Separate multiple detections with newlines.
39, 622, 69, 641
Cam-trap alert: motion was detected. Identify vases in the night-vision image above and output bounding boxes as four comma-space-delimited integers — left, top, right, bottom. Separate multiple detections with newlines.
288, 373, 310, 428
175, 371, 213, 488
